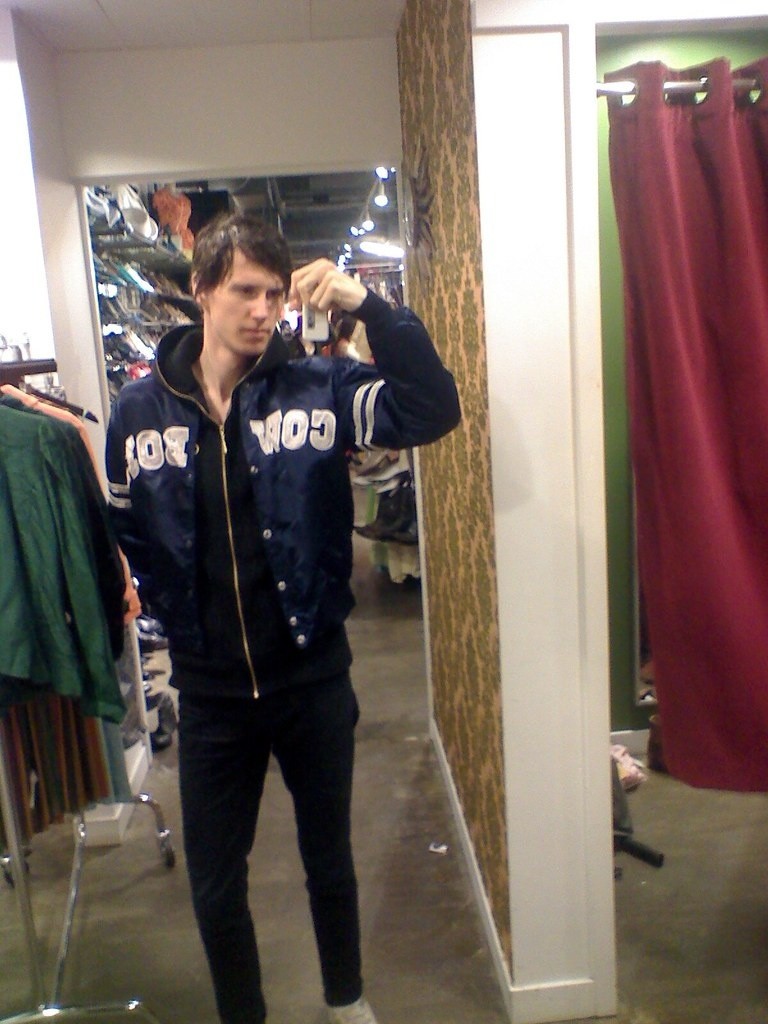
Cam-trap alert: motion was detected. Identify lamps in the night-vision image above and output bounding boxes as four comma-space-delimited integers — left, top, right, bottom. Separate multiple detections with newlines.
376, 182, 387, 206
362, 207, 374, 232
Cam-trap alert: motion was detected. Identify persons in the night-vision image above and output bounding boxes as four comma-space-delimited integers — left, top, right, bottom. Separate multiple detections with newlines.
104, 213, 461, 1024
280, 321, 306, 358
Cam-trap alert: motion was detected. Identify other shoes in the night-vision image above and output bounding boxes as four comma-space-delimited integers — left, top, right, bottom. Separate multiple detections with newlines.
323, 994, 378, 1024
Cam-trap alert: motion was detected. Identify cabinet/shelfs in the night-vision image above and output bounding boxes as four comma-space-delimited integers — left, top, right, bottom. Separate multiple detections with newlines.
89, 220, 196, 387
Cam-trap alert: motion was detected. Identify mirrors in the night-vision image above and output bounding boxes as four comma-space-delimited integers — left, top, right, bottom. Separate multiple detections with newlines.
0, 1, 768, 1024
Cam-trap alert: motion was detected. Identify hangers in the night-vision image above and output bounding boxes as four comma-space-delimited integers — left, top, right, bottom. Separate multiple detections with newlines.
18, 375, 96, 423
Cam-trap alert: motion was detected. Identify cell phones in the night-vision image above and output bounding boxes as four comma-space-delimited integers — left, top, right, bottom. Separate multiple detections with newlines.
301, 303, 330, 342
